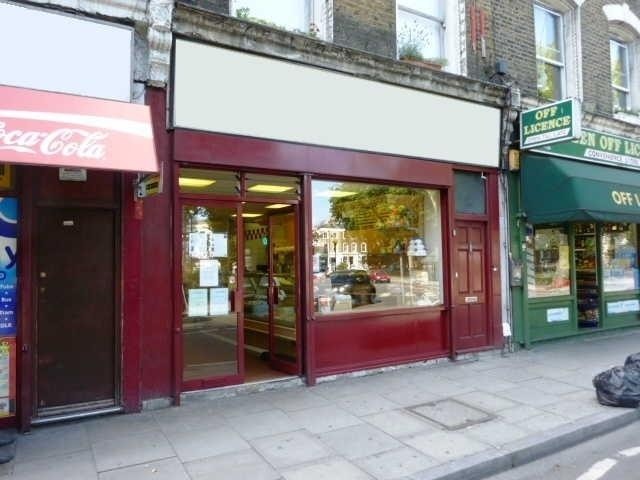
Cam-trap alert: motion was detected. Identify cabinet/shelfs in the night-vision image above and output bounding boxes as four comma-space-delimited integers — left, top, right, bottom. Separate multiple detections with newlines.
575, 222, 639, 328
534, 245, 570, 285
244, 270, 298, 368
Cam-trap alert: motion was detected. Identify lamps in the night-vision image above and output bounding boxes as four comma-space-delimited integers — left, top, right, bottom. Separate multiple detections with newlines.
489, 60, 507, 80
